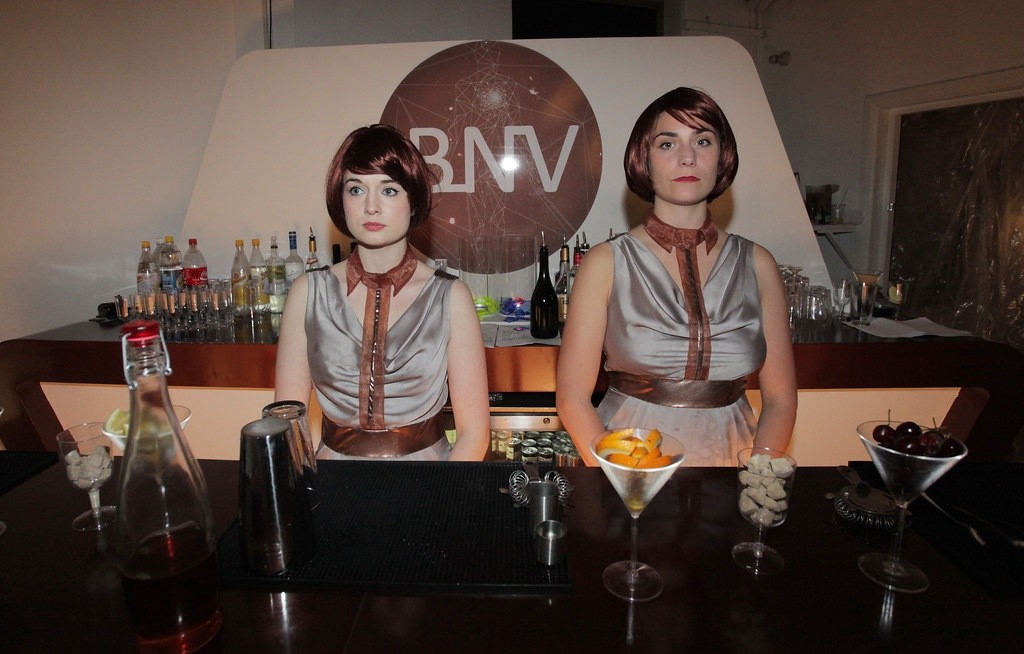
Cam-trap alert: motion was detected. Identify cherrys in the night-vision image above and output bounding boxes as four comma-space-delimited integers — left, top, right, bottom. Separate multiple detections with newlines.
873, 422, 962, 458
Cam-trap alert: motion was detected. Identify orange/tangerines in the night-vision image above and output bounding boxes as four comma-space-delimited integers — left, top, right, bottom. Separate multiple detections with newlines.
596, 428, 672, 469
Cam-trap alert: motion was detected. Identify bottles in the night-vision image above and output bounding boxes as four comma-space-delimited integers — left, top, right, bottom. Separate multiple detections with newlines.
529, 482, 567, 567
119, 320, 223, 654
553, 229, 617, 332
530, 230, 559, 339
114, 228, 341, 344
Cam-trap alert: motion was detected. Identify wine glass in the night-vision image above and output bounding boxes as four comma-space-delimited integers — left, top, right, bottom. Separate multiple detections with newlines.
101, 405, 193, 558
730, 446, 796, 576
595, 430, 682, 601
856, 421, 968, 594
55, 422, 119, 532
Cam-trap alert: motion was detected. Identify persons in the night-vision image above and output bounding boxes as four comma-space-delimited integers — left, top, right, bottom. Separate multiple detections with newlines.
272, 124, 491, 460
556, 87, 798, 467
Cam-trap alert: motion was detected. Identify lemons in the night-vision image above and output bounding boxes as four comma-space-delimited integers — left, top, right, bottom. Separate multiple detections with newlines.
889, 286, 902, 304
104, 408, 165, 439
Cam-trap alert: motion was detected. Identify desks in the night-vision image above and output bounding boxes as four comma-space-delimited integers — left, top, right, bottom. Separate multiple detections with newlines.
812, 224, 856, 270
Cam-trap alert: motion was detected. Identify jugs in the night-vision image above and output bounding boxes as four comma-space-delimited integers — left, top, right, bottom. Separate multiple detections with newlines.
804, 183, 839, 225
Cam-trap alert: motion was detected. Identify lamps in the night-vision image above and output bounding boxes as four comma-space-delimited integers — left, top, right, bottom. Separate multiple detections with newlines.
758, 44, 791, 69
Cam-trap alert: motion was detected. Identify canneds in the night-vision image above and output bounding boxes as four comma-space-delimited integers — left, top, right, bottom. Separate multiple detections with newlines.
491, 430, 583, 467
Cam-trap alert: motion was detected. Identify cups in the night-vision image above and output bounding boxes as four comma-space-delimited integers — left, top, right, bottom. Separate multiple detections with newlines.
772, 264, 915, 342
830, 203, 845, 224
238, 419, 316, 577
261, 400, 321, 511
